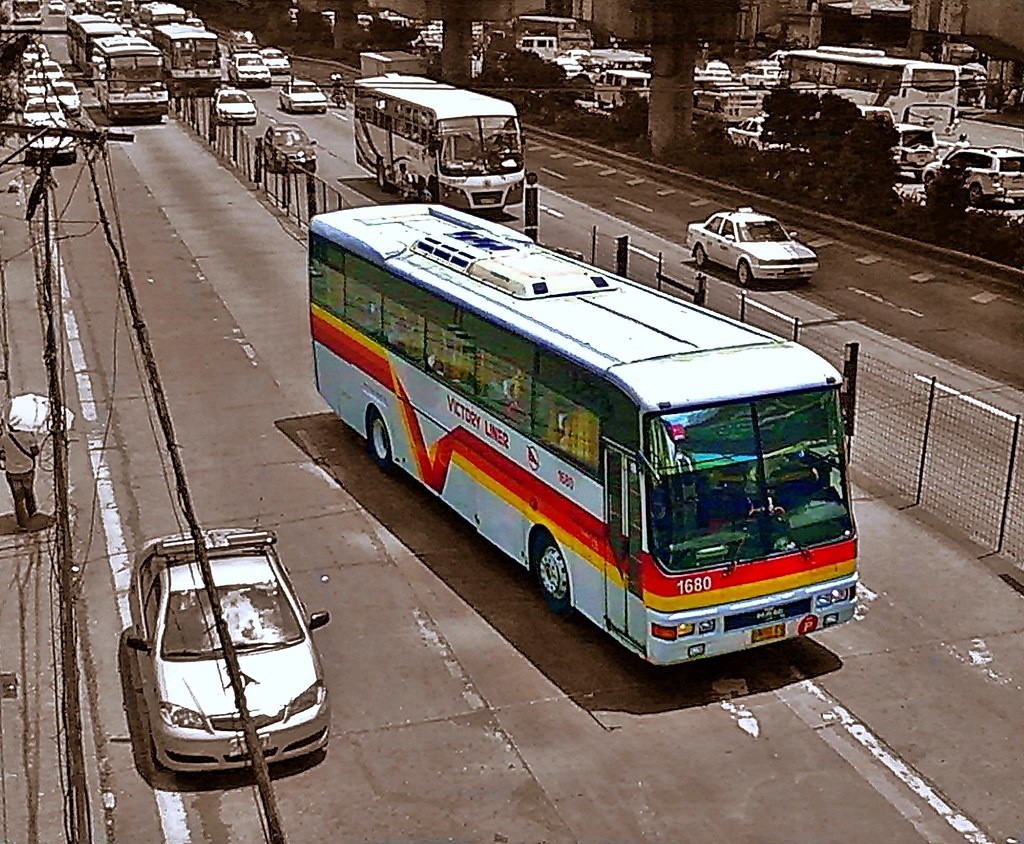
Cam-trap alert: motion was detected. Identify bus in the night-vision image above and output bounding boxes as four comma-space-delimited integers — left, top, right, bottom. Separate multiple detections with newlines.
308, 203, 859, 668
352, 75, 527, 209
762, 46, 959, 135
66, 0, 222, 123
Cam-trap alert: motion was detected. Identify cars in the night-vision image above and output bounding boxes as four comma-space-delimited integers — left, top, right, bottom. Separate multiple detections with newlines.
279, 81, 327, 113
227, 36, 291, 86
11, 0, 83, 165
125, 527, 330, 775
685, 206, 819, 287
47, 0, 67, 15
263, 124, 317, 174
214, 89, 257, 125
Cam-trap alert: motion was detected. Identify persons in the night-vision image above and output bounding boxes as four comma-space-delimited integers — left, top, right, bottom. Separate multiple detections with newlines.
0, 420, 40, 526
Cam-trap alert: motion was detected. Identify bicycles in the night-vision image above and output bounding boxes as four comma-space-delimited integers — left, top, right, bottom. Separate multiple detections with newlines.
333, 89, 346, 108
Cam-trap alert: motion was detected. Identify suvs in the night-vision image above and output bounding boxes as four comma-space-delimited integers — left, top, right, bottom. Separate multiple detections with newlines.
923, 145, 1023, 207
514, 36, 934, 181
